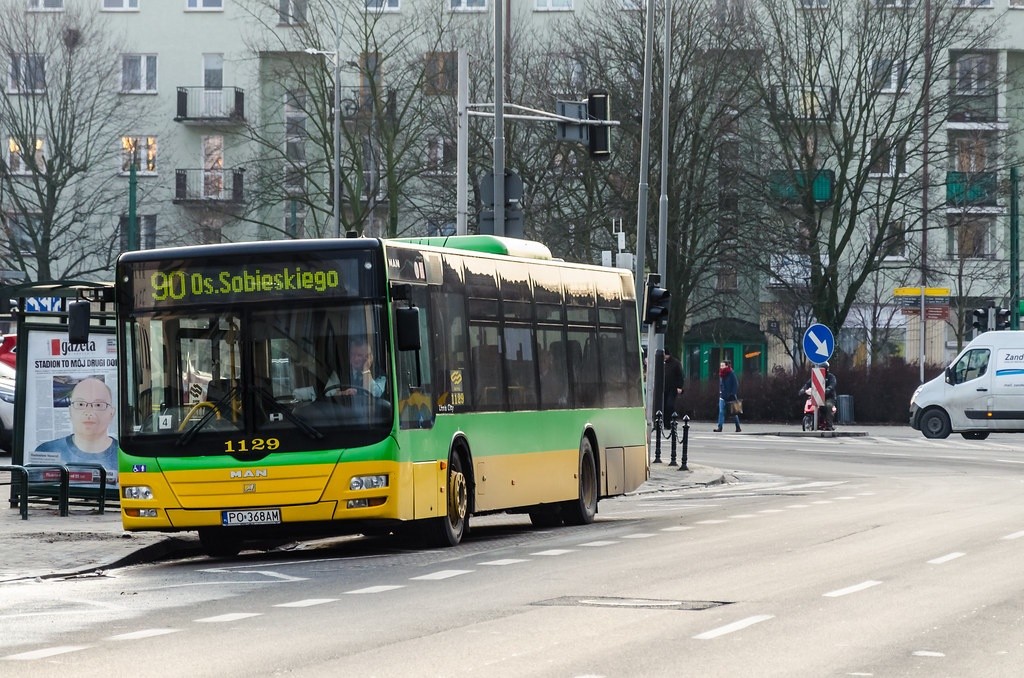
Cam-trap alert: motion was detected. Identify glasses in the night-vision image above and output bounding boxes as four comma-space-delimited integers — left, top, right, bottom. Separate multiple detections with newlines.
70, 400, 111, 411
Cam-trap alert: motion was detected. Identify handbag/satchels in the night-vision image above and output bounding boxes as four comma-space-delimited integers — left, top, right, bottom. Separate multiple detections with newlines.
730, 396, 743, 414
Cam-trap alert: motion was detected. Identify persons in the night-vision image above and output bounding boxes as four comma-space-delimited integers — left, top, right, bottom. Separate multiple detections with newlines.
713, 360, 742, 432
799, 361, 838, 431
324, 337, 386, 399
642, 346, 683, 430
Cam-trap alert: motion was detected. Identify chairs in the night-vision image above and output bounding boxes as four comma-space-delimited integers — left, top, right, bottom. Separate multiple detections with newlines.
206, 335, 637, 431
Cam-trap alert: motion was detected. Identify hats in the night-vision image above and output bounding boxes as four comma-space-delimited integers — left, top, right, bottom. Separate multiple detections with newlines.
664, 347, 672, 355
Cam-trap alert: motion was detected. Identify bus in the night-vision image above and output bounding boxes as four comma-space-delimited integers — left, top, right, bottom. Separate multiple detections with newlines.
68, 234, 651, 557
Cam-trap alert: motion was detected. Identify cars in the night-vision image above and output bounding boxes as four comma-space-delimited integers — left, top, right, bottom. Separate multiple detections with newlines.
0, 333, 18, 455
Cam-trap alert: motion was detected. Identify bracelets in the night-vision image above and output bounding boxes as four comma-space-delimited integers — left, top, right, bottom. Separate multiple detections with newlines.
361, 369, 370, 375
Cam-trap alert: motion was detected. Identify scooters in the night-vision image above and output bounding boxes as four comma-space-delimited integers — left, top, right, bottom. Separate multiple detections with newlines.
802, 387, 837, 431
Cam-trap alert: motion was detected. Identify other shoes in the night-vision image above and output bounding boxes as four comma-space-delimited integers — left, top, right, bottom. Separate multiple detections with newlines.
736, 427, 741, 432
664, 426, 671, 430
713, 428, 722, 432
825, 425, 833, 430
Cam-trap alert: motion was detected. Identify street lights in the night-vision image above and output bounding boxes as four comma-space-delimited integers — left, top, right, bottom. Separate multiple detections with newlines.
303, 47, 341, 240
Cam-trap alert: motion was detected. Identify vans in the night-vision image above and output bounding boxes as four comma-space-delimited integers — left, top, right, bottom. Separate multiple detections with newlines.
909, 330, 1024, 441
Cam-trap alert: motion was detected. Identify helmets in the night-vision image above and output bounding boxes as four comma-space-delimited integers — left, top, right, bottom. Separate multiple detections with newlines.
818, 360, 830, 368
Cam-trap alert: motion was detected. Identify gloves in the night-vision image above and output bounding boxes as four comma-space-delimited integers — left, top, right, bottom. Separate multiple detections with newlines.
825, 389, 829, 394
729, 395, 734, 400
799, 389, 805, 396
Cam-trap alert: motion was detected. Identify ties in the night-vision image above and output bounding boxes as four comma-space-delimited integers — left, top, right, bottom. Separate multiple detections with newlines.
355, 371, 362, 385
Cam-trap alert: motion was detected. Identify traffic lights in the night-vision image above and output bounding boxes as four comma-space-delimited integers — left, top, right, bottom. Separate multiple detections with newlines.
972, 307, 990, 333
642, 273, 671, 324
992, 307, 1011, 330
